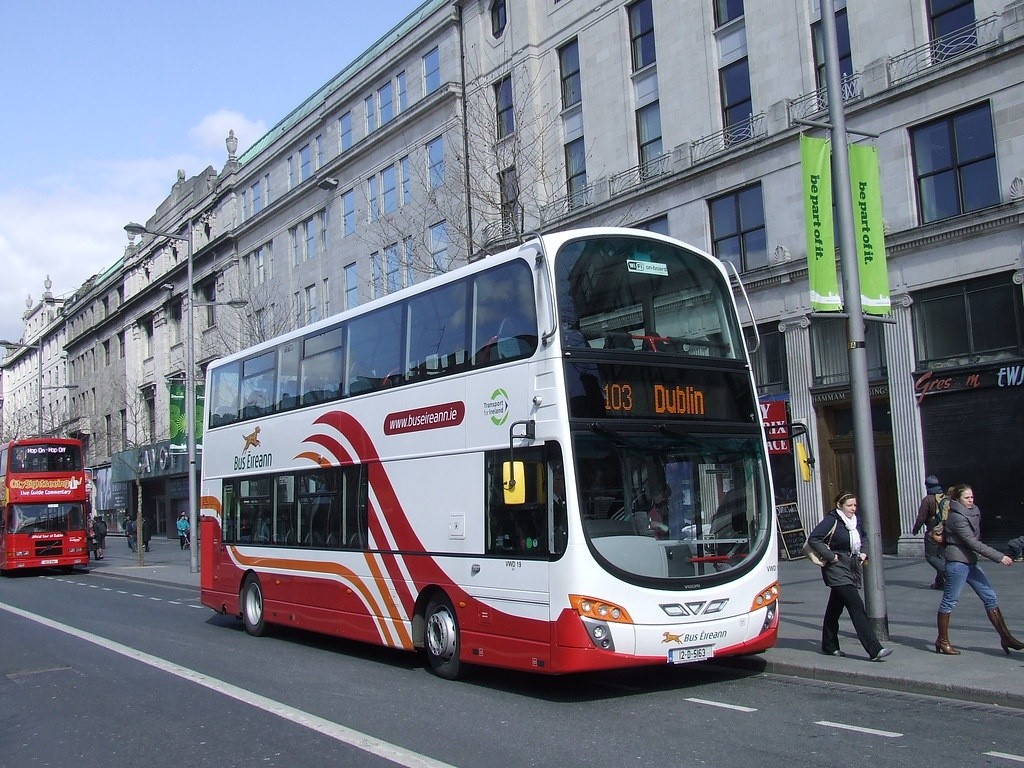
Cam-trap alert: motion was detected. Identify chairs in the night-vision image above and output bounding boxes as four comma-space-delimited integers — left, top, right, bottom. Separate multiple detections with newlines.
211, 315, 663, 547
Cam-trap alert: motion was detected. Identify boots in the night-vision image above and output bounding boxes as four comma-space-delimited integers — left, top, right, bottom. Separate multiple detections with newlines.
935, 613, 960, 655
987, 607, 1024, 654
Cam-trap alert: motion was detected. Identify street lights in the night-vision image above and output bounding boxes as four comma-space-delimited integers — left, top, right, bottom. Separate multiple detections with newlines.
0, 336, 79, 440
124, 218, 249, 573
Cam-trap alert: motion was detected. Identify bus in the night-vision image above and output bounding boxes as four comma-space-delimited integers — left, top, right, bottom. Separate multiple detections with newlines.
198, 226, 815, 682
0, 438, 91, 571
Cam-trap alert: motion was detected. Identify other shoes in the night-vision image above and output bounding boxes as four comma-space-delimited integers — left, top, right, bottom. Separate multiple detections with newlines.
96, 556, 103, 560
931, 584, 944, 590
823, 650, 846, 657
872, 648, 894, 661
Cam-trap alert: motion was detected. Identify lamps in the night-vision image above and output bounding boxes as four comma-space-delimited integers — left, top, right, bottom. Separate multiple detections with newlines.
161, 284, 175, 292
317, 177, 338, 190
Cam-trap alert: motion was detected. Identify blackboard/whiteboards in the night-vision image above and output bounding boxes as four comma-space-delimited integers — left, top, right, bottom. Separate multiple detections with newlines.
775, 502, 807, 561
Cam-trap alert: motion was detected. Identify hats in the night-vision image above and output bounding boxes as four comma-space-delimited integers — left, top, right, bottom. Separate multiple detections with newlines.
925, 475, 939, 487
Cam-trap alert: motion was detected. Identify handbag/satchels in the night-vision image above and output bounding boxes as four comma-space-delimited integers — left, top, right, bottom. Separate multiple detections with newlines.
803, 520, 838, 566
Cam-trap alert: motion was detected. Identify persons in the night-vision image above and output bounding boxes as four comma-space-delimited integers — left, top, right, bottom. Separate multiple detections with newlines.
934, 484, 1024, 655
706, 506, 733, 571
88, 516, 107, 560
607, 483, 671, 537
912, 476, 946, 589
1008, 536, 1024, 561
142, 517, 151, 552
177, 511, 190, 550
808, 491, 893, 661
534, 470, 566, 528
123, 515, 137, 553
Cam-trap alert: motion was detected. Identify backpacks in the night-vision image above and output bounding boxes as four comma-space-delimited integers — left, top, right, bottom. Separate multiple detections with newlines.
935, 493, 950, 520
97, 523, 107, 535
